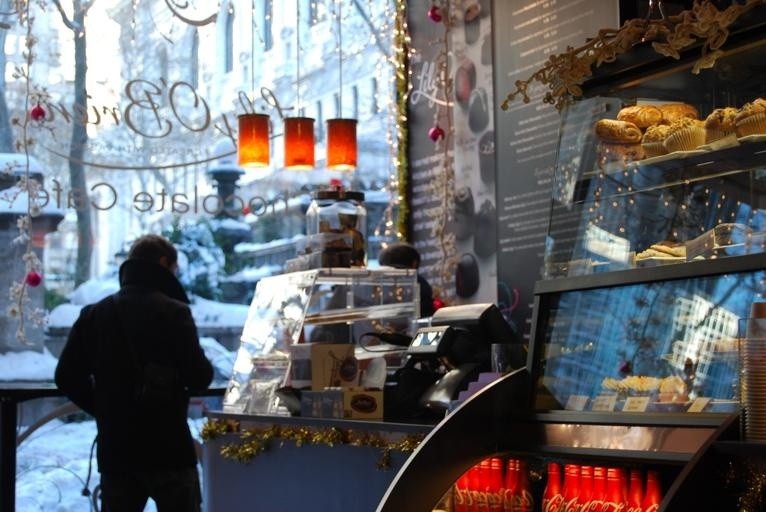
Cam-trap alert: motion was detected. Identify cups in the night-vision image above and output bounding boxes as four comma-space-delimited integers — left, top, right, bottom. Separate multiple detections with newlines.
462, 5, 480, 46
473, 202, 498, 260
742, 301, 766, 441
455, 60, 477, 101
454, 187, 474, 239
481, 34, 492, 65
456, 252, 479, 298
479, 131, 496, 186
467, 86, 489, 133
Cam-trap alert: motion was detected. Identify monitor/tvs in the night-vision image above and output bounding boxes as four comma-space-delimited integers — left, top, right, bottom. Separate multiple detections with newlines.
431, 303, 528, 370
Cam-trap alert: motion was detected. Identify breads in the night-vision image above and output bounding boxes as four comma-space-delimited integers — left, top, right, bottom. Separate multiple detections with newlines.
594, 95, 766, 170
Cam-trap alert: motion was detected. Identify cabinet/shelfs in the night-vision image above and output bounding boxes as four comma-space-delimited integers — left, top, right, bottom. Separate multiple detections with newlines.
373, 22, 766, 512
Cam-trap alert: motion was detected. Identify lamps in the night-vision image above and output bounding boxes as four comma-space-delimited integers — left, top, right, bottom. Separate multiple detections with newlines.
283, 0, 315, 170
237, 0, 270, 168
325, 0, 358, 172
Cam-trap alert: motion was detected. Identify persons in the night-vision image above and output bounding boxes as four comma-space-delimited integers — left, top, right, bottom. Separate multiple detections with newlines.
54, 234, 215, 511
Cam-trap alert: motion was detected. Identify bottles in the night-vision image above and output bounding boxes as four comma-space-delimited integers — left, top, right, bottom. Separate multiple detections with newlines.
450, 457, 663, 511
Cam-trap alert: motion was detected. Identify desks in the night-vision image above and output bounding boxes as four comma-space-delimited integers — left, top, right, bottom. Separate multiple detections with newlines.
0, 379, 227, 512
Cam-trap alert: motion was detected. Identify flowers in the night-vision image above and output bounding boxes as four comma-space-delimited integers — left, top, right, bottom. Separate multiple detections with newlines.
9, 1, 53, 347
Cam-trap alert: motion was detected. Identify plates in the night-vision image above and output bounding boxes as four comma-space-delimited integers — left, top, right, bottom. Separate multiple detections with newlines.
590, 397, 742, 410
580, 133, 766, 175
590, 241, 744, 270
